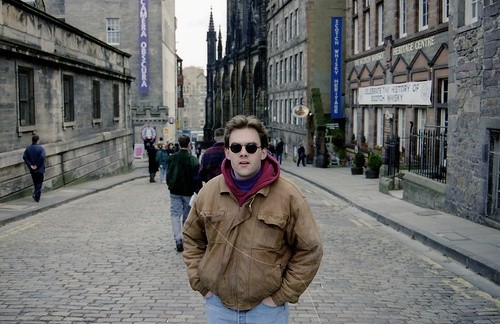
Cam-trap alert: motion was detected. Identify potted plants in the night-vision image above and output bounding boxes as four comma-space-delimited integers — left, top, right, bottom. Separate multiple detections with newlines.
351, 150, 366, 175
365, 153, 383, 178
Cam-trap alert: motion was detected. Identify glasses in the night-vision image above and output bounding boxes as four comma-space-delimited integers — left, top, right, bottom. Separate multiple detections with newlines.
229, 143, 261, 154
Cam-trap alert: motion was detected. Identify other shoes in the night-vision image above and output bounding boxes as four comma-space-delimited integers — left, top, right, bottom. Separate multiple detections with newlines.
35, 198, 39, 202
33, 194, 36, 198
177, 244, 183, 251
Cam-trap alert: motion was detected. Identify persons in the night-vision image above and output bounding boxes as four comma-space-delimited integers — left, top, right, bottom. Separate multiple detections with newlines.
143, 137, 201, 183
23, 135, 45, 202
297, 143, 306, 167
268, 138, 284, 165
183, 116, 323, 324
166, 128, 226, 252
